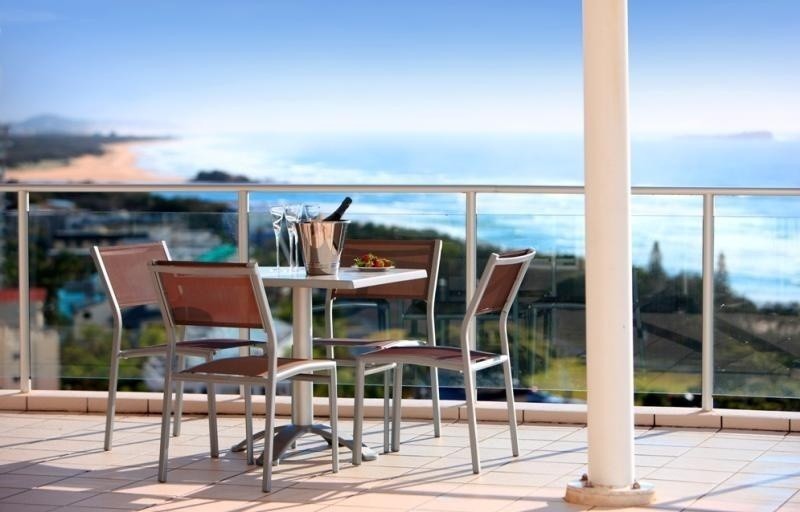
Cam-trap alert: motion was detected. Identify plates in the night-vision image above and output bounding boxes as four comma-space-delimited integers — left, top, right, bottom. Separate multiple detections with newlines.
350, 264, 394, 271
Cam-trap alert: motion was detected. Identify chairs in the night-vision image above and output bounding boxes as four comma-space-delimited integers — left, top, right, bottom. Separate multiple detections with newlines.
92, 240, 272, 459
353, 249, 536, 474
292, 234, 443, 453
145, 260, 338, 490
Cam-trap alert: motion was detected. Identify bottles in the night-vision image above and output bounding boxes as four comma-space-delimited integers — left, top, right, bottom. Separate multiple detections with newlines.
323, 196, 352, 220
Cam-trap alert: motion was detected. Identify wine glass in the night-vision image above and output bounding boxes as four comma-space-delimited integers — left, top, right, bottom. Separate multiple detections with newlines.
267, 202, 321, 274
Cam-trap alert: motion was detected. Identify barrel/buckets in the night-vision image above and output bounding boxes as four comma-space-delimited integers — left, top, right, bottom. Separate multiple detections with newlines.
292, 220, 350, 275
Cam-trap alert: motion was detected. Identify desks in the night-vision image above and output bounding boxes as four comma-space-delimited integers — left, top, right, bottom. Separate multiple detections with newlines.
230, 265, 427, 468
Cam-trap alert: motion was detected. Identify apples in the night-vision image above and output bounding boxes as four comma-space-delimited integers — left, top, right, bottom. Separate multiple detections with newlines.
357, 255, 391, 267
